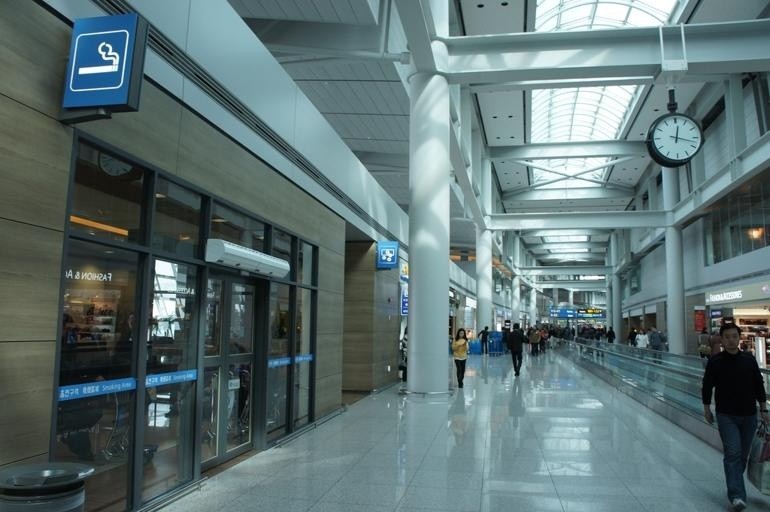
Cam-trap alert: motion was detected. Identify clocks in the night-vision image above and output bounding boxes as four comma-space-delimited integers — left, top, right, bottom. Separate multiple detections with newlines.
646, 109, 705, 169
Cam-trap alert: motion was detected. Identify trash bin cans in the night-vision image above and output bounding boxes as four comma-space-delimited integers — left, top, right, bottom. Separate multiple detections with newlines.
0, 462, 95, 512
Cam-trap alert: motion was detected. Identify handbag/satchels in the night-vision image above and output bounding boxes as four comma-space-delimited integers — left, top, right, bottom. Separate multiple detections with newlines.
746, 419, 770, 497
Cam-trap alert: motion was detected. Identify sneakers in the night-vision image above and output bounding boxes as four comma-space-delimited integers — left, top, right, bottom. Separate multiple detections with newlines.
730, 496, 746, 512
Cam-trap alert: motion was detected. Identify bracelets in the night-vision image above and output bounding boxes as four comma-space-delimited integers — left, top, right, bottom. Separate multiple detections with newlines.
759, 409, 768, 413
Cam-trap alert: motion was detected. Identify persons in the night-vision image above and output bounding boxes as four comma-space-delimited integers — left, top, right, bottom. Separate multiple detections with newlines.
509, 378, 526, 428
505, 322, 530, 378
527, 320, 604, 357
701, 323, 770, 510
448, 389, 469, 445
477, 355, 490, 385
628, 325, 669, 365
61, 299, 284, 461
697, 326, 713, 359
710, 331, 722, 355
451, 328, 469, 389
477, 326, 489, 355
527, 357, 578, 392
605, 327, 616, 343
